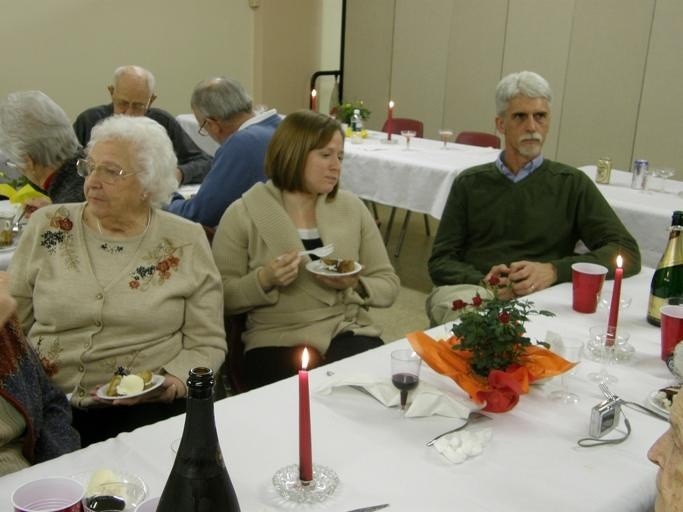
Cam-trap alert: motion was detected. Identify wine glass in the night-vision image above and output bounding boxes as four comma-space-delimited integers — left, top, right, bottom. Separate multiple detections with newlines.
546, 326, 631, 404
438, 129, 453, 149
597, 291, 632, 324
392, 349, 422, 413
401, 131, 416, 152
639, 166, 677, 195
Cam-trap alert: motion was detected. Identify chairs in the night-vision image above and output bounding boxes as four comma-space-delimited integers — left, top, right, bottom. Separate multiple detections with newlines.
361, 118, 424, 246
392, 131, 501, 258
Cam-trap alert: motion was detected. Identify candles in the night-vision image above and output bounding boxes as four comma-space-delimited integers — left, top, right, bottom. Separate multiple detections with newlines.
387, 102, 393, 140
607, 255, 624, 346
298, 346, 313, 482
311, 89, 317, 113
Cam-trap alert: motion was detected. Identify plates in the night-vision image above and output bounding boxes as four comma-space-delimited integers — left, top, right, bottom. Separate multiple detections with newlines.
305, 259, 362, 276
648, 356, 683, 414
97, 374, 166, 399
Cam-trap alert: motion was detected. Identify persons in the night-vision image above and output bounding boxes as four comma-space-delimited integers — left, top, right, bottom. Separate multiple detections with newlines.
70, 63, 221, 189
0, 271, 87, 481
421, 69, 644, 339
1, 108, 233, 446
646, 340, 683, 512
1, 89, 95, 216
208, 108, 403, 390
157, 73, 285, 243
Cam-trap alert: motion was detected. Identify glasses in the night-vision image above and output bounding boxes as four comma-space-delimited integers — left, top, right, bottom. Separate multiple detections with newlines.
2, 159, 20, 169
75, 158, 147, 186
196, 119, 209, 137
111, 91, 151, 112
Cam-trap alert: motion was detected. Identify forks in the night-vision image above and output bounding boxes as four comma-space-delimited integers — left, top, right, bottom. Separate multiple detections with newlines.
599, 384, 670, 424
278, 244, 335, 260
427, 412, 492, 446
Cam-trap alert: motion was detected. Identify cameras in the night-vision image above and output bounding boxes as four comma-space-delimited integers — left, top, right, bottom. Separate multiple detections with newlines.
590, 396, 621, 438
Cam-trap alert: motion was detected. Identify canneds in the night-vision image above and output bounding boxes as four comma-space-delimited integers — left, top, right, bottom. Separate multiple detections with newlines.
631, 160, 648, 190
596, 158, 611, 184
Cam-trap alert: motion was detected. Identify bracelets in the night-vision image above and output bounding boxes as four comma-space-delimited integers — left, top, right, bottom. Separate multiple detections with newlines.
170, 382, 180, 402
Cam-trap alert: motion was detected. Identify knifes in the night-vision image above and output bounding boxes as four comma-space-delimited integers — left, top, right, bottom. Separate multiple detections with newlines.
347, 505, 389, 512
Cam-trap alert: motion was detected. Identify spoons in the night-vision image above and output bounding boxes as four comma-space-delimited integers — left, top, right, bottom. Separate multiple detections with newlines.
12, 197, 36, 232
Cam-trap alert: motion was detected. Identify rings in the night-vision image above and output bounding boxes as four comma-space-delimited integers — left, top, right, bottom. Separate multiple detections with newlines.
530, 284, 535, 290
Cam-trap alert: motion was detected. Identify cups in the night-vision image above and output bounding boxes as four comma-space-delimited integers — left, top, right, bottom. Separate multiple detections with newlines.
10, 472, 161, 512
660, 306, 683, 362
0, 212, 16, 250
571, 263, 608, 313
170, 438, 182, 463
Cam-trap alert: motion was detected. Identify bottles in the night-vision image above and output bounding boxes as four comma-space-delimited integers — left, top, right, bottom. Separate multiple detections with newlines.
647, 211, 683, 327
156, 367, 241, 512
351, 110, 362, 144
